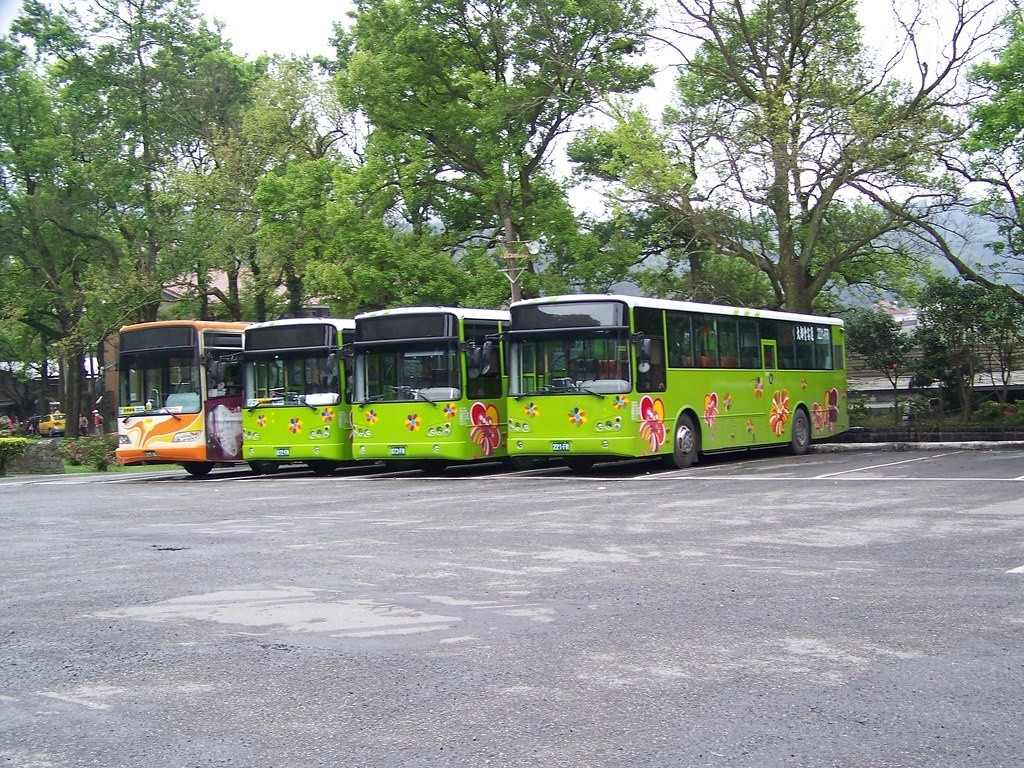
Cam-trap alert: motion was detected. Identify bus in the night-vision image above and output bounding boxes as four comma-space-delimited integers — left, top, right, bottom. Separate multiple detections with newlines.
344, 305, 536, 469
220, 317, 359, 475
94, 318, 266, 476
480, 294, 850, 473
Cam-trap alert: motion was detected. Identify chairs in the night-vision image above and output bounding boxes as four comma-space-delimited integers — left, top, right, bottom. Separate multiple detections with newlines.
432, 368, 449, 388
570, 351, 665, 390
670, 354, 832, 370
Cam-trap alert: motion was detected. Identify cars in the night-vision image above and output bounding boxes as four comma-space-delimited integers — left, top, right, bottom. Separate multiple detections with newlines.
33, 410, 67, 436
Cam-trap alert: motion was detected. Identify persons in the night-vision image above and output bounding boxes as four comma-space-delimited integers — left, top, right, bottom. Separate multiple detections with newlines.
79, 413, 90, 437
92, 410, 103, 435
33, 414, 41, 437
